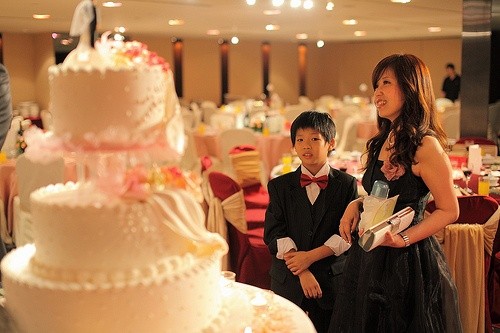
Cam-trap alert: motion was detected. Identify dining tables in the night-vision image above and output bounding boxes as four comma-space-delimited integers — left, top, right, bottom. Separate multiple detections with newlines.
268, 156, 500, 330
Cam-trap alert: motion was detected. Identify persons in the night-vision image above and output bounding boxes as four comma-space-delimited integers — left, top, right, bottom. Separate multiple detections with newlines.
262, 111, 356, 333
330, 53, 461, 333
440, 62, 460, 103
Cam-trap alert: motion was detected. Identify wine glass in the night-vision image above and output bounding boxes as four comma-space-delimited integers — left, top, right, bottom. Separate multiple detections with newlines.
461, 162, 474, 191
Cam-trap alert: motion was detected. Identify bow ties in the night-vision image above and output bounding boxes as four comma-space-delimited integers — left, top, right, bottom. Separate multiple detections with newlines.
300, 173, 328, 190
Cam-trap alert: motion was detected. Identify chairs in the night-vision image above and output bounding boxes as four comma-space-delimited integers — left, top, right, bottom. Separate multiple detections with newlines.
181, 93, 500, 333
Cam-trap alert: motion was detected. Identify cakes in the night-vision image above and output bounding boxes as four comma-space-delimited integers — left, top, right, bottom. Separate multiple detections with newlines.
0, 163, 229, 333
47, 0, 169, 152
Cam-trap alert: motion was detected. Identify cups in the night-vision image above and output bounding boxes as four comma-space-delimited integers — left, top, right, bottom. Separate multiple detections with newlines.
465, 140, 474, 150
262, 123, 269, 136
282, 153, 291, 174
478, 177, 490, 196
339, 163, 347, 171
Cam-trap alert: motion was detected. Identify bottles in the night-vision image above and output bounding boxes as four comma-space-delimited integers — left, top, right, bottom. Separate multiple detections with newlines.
358, 181, 388, 232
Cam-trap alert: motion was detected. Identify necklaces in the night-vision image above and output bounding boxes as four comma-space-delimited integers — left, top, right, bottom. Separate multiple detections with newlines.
387, 127, 403, 145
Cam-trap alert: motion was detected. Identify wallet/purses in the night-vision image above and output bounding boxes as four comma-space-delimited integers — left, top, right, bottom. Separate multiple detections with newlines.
357, 206, 415, 253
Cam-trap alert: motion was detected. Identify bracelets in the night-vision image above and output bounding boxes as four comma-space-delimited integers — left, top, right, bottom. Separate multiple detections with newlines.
398, 232, 410, 247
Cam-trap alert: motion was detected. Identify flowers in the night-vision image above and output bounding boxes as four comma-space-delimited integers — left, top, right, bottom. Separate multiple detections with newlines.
381, 159, 405, 181
